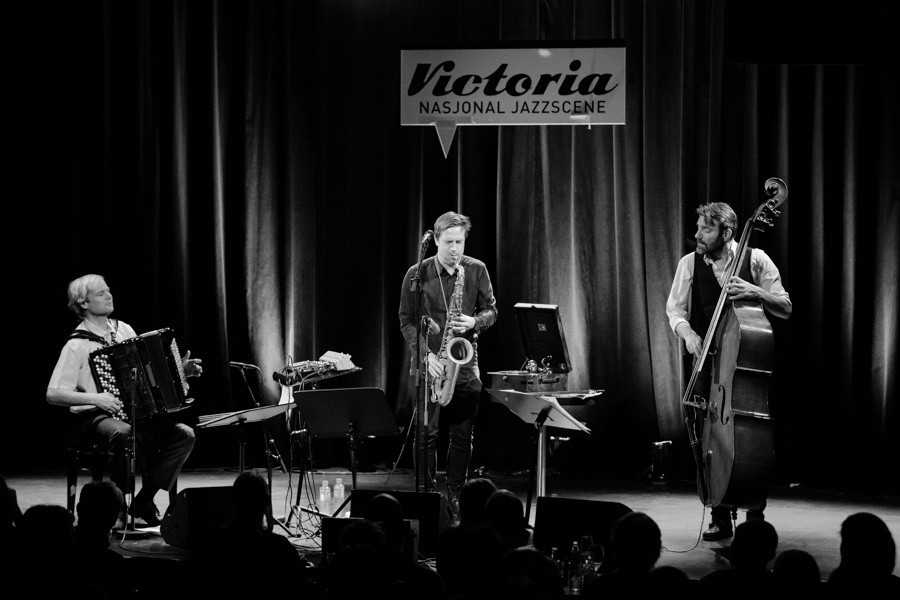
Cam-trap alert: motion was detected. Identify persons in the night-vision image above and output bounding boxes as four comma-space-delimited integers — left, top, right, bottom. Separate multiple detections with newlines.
398, 211, 498, 513
47, 275, 203, 526
666, 203, 792, 541
0, 471, 900, 600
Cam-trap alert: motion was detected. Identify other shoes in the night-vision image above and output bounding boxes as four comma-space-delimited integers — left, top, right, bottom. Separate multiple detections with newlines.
703, 525, 733, 541
449, 492, 460, 513
128, 497, 161, 526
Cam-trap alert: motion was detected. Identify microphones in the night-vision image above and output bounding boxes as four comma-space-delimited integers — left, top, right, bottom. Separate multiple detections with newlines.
229, 361, 255, 369
421, 230, 434, 245
422, 316, 440, 335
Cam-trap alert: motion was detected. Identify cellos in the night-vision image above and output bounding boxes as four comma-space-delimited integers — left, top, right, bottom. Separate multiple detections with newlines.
680, 177, 788, 534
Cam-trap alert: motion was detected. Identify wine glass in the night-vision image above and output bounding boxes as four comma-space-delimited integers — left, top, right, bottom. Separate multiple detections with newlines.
580, 537, 605, 576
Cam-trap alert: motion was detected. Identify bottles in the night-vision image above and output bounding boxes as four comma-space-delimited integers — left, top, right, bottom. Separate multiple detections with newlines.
568, 541, 583, 592
332, 478, 344, 519
551, 547, 561, 574
319, 480, 331, 517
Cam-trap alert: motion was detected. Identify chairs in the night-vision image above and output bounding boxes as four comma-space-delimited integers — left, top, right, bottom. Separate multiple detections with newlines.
54, 410, 177, 532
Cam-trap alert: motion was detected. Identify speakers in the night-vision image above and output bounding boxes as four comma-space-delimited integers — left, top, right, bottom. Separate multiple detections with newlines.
350, 488, 457, 562
160, 486, 234, 550
533, 496, 634, 562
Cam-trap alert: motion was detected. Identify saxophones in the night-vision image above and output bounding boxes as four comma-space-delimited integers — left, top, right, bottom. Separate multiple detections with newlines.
428, 255, 474, 408
